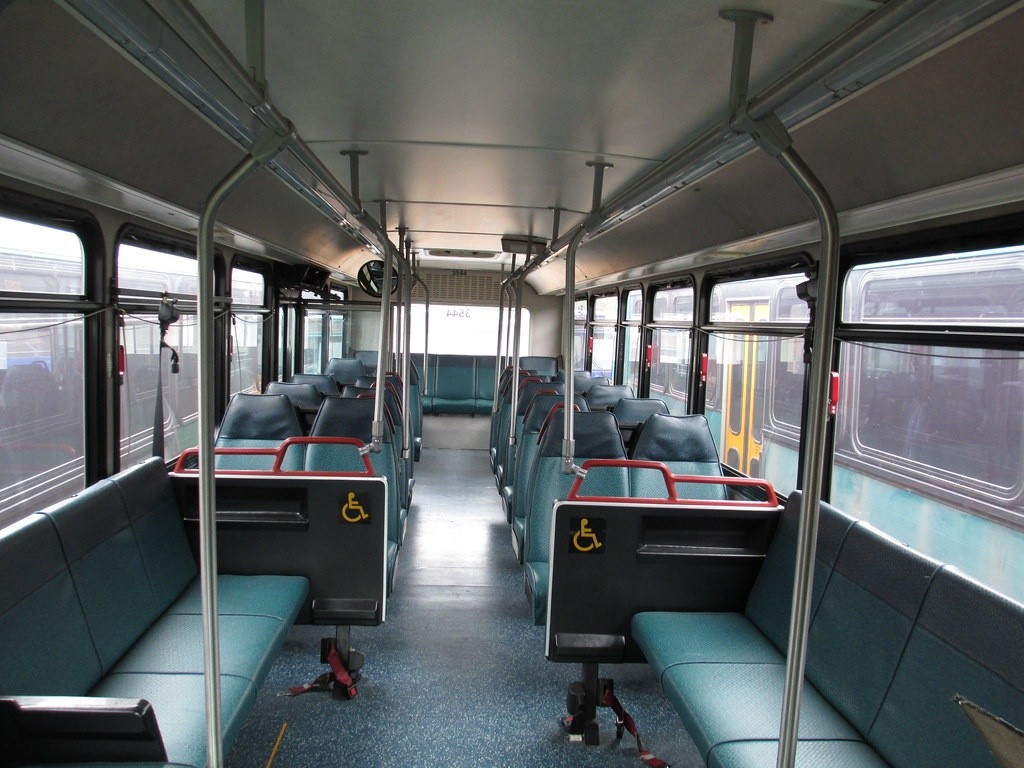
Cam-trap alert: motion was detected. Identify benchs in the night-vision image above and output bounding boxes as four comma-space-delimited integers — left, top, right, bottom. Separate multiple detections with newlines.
215, 351, 726, 627
0, 457, 311, 768
632, 489, 1024, 768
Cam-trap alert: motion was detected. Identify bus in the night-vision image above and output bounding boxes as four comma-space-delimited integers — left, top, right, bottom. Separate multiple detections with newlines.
603, 245, 1023, 612
1, 249, 344, 531
